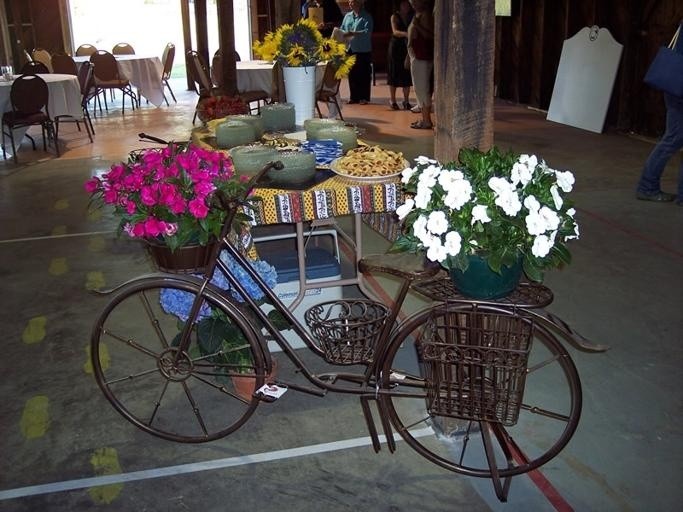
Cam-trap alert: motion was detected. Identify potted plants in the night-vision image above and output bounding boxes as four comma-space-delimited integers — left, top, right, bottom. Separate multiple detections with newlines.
443, 250, 525, 302
145, 237, 217, 275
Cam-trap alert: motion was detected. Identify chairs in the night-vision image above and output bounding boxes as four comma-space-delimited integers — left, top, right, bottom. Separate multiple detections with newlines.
23, 40, 178, 117
185, 40, 349, 126
0, 41, 95, 165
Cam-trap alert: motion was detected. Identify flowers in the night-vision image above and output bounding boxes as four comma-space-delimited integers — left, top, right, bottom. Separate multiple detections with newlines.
157, 254, 277, 371
391, 143, 584, 282
83, 139, 265, 252
251, 17, 357, 80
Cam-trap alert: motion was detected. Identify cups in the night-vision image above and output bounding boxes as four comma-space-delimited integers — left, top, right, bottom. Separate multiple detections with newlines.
0, 65, 14, 81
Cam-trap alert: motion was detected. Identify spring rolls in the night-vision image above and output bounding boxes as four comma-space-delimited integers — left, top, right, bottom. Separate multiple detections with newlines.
335, 139, 403, 177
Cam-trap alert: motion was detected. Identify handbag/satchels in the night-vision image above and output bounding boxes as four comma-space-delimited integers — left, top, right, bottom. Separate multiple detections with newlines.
643, 46, 683, 98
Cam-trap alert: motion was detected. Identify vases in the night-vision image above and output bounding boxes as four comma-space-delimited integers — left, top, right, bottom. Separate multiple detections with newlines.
230, 360, 278, 403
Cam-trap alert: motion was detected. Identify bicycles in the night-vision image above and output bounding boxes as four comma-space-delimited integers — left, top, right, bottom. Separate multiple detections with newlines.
90, 156, 612, 502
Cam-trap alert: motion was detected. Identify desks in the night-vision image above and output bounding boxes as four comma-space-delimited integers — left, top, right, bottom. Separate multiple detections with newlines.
186, 120, 415, 360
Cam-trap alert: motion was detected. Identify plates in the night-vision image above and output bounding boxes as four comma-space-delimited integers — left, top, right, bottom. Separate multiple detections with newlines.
329, 153, 410, 183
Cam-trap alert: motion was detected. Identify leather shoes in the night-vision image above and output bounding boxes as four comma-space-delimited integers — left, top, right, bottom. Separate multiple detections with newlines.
638, 191, 674, 202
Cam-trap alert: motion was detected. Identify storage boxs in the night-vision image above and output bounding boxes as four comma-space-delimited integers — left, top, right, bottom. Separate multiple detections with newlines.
237, 245, 343, 357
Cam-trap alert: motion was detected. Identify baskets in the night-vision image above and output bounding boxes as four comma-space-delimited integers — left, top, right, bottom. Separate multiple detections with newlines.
304, 299, 388, 365
415, 309, 535, 425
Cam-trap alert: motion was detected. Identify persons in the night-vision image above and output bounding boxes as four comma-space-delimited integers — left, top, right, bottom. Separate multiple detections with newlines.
301, 1, 316, 24
338, 0, 374, 105
406, 0, 433, 128
385, 0, 411, 112
634, 23, 683, 205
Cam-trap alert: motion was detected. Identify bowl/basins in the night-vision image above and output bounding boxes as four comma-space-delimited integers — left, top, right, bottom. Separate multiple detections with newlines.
206, 98, 358, 188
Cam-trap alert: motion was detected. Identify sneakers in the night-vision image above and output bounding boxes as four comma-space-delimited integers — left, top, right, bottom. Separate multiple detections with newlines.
349, 98, 434, 128
